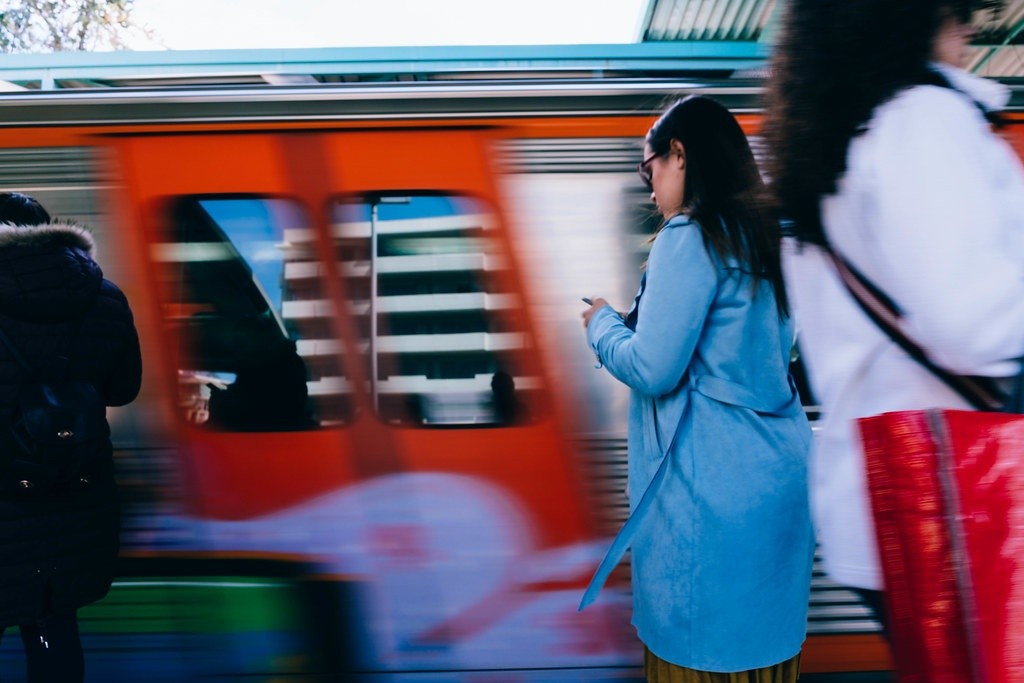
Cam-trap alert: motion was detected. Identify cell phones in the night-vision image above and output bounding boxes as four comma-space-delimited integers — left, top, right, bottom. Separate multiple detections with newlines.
582, 298, 594, 306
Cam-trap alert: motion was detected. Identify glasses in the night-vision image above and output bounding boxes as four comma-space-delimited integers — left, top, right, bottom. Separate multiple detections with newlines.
638, 146, 669, 193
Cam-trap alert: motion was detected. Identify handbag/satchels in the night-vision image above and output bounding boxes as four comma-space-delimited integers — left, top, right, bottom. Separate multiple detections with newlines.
789, 355, 822, 421
856, 407, 1024, 683
945, 369, 1024, 410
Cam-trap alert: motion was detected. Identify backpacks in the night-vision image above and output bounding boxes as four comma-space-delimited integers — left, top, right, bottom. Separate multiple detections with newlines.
0, 318, 111, 497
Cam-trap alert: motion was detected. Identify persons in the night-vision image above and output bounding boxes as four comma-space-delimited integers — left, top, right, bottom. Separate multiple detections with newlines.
0, 193, 141, 683
762, 0, 1024, 657
582, 96, 813, 683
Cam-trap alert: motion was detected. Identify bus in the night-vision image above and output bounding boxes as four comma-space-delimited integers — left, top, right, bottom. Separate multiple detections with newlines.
1, 77, 1024, 681
